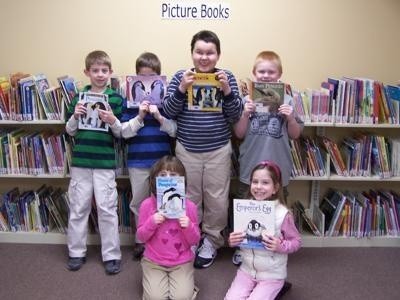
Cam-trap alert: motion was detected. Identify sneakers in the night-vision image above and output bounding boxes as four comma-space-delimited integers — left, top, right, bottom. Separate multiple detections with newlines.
194, 237, 217, 268
104, 260, 121, 274
133, 243, 143, 259
232, 250, 243, 266
68, 257, 87, 270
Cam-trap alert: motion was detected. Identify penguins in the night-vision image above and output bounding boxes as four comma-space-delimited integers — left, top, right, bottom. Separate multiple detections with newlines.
195, 87, 207, 107
246, 219, 262, 243
209, 86, 221, 107
151, 79, 164, 104
95, 102, 106, 128
162, 188, 176, 209
89, 104, 97, 128
131, 81, 146, 104
166, 193, 182, 215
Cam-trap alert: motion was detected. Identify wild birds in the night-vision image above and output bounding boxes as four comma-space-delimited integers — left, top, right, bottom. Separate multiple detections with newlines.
255, 87, 283, 118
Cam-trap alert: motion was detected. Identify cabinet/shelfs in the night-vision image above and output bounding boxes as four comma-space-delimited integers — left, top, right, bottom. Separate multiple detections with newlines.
1, 114, 400, 250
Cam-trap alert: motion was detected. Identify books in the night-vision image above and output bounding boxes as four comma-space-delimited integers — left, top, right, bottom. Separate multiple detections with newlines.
228, 74, 399, 237
230, 196, 277, 250
0, 73, 128, 233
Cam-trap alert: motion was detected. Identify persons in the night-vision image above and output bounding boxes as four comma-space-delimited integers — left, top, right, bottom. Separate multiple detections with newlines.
63, 49, 129, 275
119, 51, 178, 257
160, 29, 245, 268
227, 50, 305, 266
223, 158, 303, 300
133, 152, 202, 300
